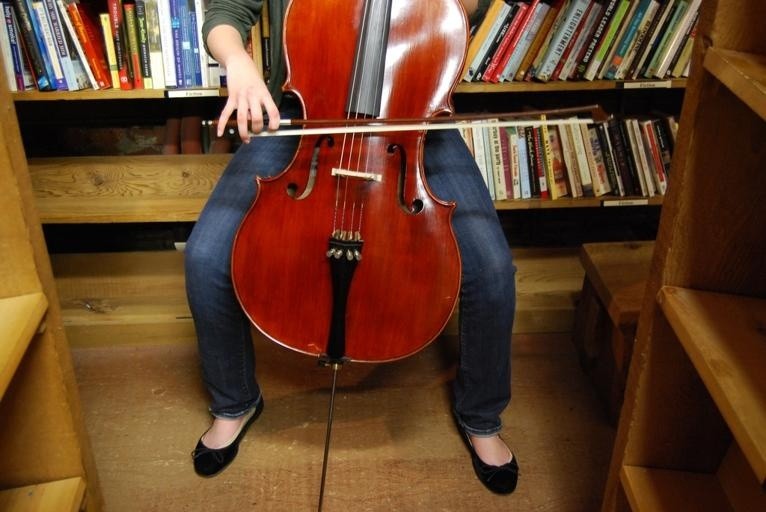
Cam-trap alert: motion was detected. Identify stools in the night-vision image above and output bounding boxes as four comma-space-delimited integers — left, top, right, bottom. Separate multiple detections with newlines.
570, 236, 652, 433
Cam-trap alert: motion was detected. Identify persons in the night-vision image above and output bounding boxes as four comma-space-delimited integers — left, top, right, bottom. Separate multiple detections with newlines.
181, 0, 521, 496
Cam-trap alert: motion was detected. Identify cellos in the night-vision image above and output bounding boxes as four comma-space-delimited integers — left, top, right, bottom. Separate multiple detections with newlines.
231, 1, 470, 365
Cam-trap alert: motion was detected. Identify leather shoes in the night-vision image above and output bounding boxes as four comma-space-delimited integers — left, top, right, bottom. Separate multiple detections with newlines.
192, 389, 264, 480
451, 408, 518, 494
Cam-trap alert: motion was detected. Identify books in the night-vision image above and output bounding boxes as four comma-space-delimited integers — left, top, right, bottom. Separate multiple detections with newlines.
456, 1, 703, 84
1, 1, 288, 91
456, 111, 679, 202
164, 117, 236, 157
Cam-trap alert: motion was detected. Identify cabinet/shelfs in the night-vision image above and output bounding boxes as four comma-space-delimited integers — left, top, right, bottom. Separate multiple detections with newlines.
0, 50, 108, 511
597, 0, 764, 510
0, 2, 715, 219
